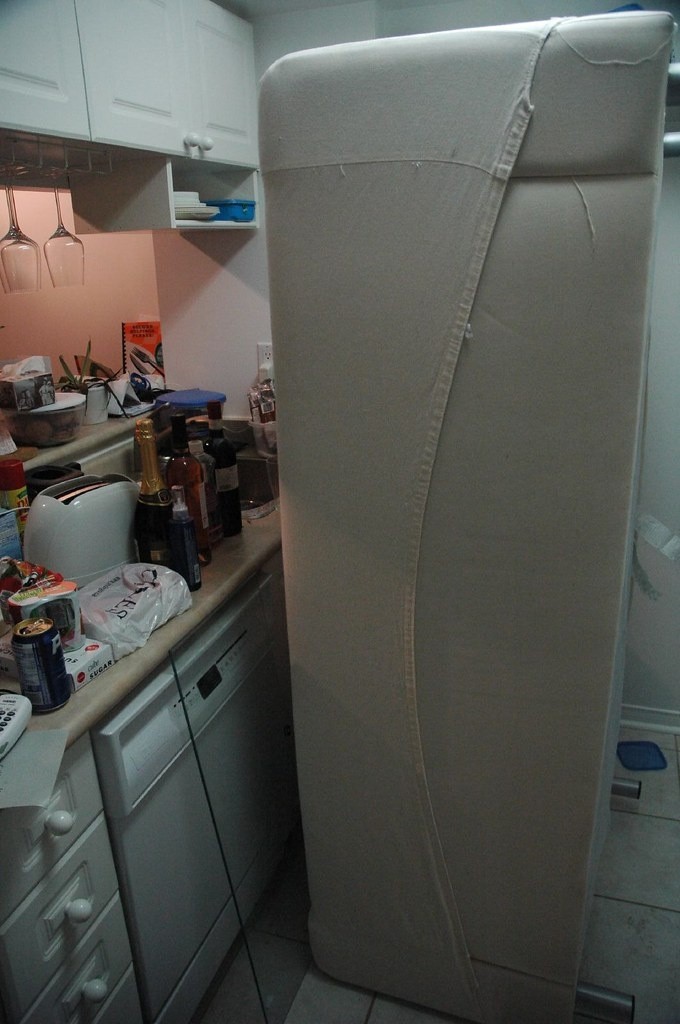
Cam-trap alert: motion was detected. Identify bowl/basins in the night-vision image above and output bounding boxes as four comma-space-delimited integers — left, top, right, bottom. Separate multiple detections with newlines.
1, 401, 85, 446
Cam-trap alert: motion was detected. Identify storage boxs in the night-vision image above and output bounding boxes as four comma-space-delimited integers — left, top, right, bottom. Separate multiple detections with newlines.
0, 392, 88, 448
248, 420, 278, 457
200, 199, 257, 223
156, 389, 226, 435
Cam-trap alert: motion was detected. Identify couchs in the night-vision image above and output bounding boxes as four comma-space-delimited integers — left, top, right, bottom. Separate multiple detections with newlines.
258, 7, 680, 1022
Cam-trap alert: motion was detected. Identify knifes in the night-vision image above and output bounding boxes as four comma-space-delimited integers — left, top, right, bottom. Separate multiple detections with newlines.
130, 354, 149, 374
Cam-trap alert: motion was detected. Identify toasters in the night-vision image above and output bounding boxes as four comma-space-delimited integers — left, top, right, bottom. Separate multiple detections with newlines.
24, 472, 140, 590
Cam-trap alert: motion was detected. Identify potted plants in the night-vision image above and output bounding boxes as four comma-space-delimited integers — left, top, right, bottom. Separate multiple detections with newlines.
52, 338, 129, 426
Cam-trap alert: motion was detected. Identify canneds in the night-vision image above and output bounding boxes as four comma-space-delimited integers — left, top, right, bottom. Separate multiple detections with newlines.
10, 616, 72, 714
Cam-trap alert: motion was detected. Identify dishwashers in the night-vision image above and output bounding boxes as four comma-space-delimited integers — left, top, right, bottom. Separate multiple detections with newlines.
89, 572, 297, 1024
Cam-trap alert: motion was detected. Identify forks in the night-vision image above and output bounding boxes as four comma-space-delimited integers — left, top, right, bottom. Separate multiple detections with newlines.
131, 347, 164, 376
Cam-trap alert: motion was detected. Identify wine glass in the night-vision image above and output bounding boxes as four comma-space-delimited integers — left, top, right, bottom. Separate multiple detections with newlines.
0, 168, 40, 293
39, 166, 85, 288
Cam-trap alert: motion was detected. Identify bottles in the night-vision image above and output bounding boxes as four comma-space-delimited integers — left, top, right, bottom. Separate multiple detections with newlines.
165, 413, 212, 566
130, 417, 175, 567
205, 400, 242, 537
188, 440, 222, 544
169, 486, 202, 591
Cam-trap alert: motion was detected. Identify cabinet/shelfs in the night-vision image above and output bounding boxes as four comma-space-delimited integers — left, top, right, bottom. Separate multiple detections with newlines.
68, 0, 261, 234
0, 730, 146, 1024
1, 0, 90, 194
